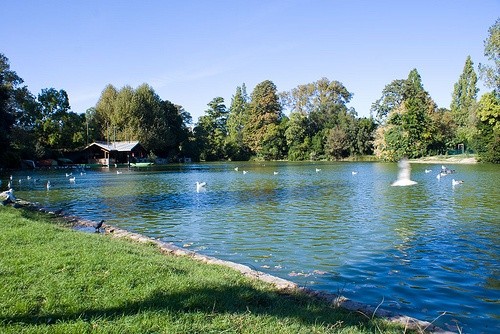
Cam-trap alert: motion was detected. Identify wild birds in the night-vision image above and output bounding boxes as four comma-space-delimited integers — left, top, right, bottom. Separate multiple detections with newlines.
352, 170, 359, 175
452, 179, 460, 185
243, 171, 248, 175
316, 167, 321, 173
53, 208, 64, 216
116, 171, 122, 174
425, 169, 432, 173
436, 164, 456, 179
195, 181, 206, 187
274, 171, 279, 175
0, 188, 16, 206
234, 167, 238, 171
389, 155, 418, 187
92, 219, 107, 232
7, 171, 87, 189
